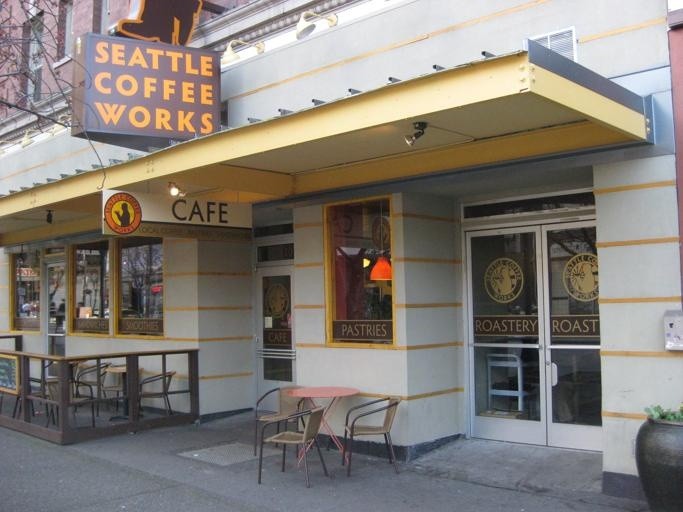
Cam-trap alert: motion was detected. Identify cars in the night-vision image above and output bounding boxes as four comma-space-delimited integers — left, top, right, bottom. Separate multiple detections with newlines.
29, 299, 143, 319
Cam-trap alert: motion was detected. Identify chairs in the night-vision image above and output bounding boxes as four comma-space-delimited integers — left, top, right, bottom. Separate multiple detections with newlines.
11, 361, 175, 429
253, 386, 328, 488
342, 395, 402, 476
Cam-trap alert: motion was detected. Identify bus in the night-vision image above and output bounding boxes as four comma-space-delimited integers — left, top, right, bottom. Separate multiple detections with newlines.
141, 280, 162, 318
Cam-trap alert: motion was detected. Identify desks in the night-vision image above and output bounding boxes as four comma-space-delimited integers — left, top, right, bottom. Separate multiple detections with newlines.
286, 386, 360, 467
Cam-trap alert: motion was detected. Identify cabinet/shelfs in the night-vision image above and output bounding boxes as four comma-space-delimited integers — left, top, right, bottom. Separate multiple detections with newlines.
485, 352, 539, 411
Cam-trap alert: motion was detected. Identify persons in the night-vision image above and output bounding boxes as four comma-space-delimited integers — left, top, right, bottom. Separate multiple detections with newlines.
17, 299, 84, 323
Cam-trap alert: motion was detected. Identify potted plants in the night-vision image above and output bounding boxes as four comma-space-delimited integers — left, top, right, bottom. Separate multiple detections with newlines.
633, 403, 682, 512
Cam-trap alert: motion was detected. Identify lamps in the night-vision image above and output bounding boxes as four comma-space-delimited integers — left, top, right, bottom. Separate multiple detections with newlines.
402, 123, 431, 149
294, 10, 337, 42
218, 36, 264, 65
370, 201, 393, 282
